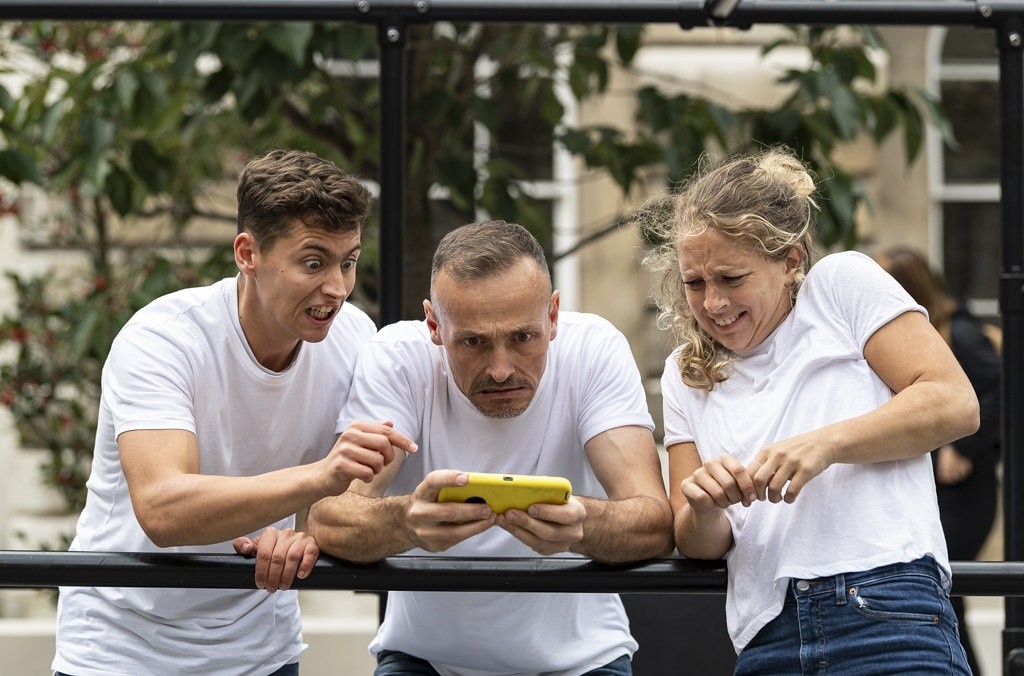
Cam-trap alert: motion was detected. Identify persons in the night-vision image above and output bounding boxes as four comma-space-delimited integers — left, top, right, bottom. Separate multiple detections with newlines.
304, 222, 674, 675
46, 150, 417, 676
647, 149, 984, 676
875, 247, 1005, 676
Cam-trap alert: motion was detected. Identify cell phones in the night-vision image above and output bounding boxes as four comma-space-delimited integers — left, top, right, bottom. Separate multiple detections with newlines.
436, 471, 573, 525
